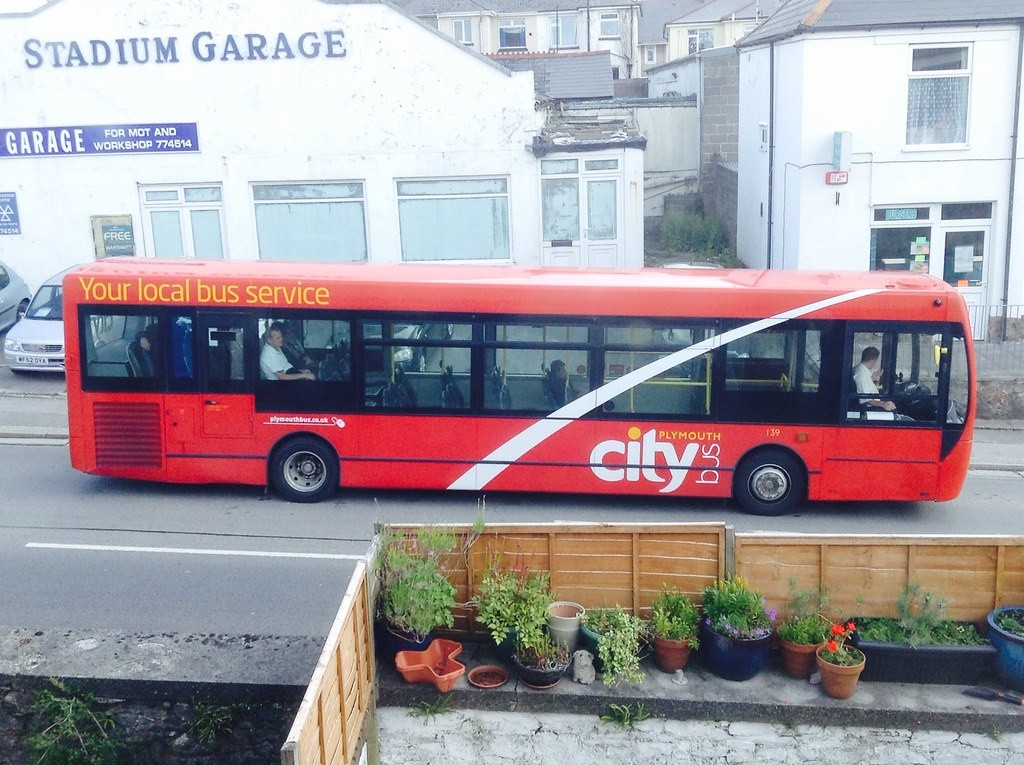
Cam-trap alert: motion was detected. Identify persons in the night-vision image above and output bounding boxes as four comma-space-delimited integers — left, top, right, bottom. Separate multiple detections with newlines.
852, 346, 896, 411
260, 321, 319, 381
136, 316, 188, 377
549, 359, 583, 407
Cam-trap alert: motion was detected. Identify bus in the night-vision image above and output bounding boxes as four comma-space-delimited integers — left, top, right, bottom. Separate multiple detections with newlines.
62, 255, 977, 517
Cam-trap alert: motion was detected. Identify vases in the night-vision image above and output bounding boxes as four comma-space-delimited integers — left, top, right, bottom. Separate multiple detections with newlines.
395, 639, 465, 692
702, 623, 774, 682
380, 626, 436, 658
545, 601, 586, 651
815, 644, 866, 699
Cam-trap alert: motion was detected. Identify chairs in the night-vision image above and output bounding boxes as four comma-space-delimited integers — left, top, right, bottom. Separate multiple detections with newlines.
183, 332, 192, 378
847, 376, 897, 414
446, 383, 464, 408
396, 384, 413, 407
564, 374, 573, 405
540, 378, 560, 410
447, 364, 468, 406
234, 332, 266, 380
540, 363, 553, 391
393, 397, 407, 408
395, 368, 415, 407
442, 390, 456, 408
499, 369, 511, 410
126, 341, 156, 377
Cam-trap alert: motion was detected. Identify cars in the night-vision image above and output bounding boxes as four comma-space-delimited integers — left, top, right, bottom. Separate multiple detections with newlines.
4, 264, 113, 374
0, 259, 32, 331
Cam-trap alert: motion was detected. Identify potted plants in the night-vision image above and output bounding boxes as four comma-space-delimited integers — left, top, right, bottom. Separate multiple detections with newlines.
776, 576, 831, 679
845, 580, 998, 687
470, 531, 559, 666
986, 606, 1024, 693
511, 626, 573, 689
643, 579, 702, 674
581, 588, 655, 689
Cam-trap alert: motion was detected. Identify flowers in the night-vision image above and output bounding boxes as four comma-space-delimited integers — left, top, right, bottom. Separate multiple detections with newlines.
699, 570, 777, 639
821, 622, 864, 666
374, 494, 486, 639
